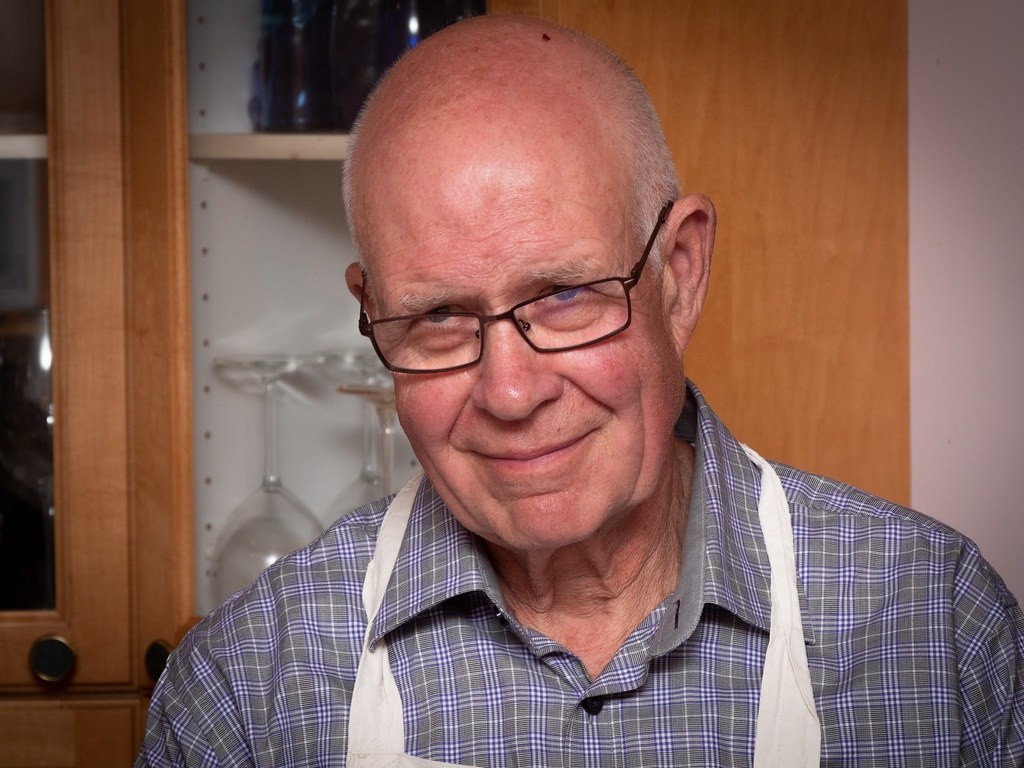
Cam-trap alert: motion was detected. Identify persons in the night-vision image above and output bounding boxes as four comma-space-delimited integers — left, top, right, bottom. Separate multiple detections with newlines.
133, 11, 1024, 768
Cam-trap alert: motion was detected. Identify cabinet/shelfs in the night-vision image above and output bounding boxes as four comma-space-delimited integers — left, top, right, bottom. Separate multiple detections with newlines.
2, 0, 910, 764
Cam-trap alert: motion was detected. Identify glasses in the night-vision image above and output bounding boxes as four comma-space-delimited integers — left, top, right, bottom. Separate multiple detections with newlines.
359, 201, 675, 373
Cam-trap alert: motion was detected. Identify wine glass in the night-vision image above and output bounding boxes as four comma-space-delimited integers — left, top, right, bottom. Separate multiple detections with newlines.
304, 350, 402, 529
208, 349, 325, 611
